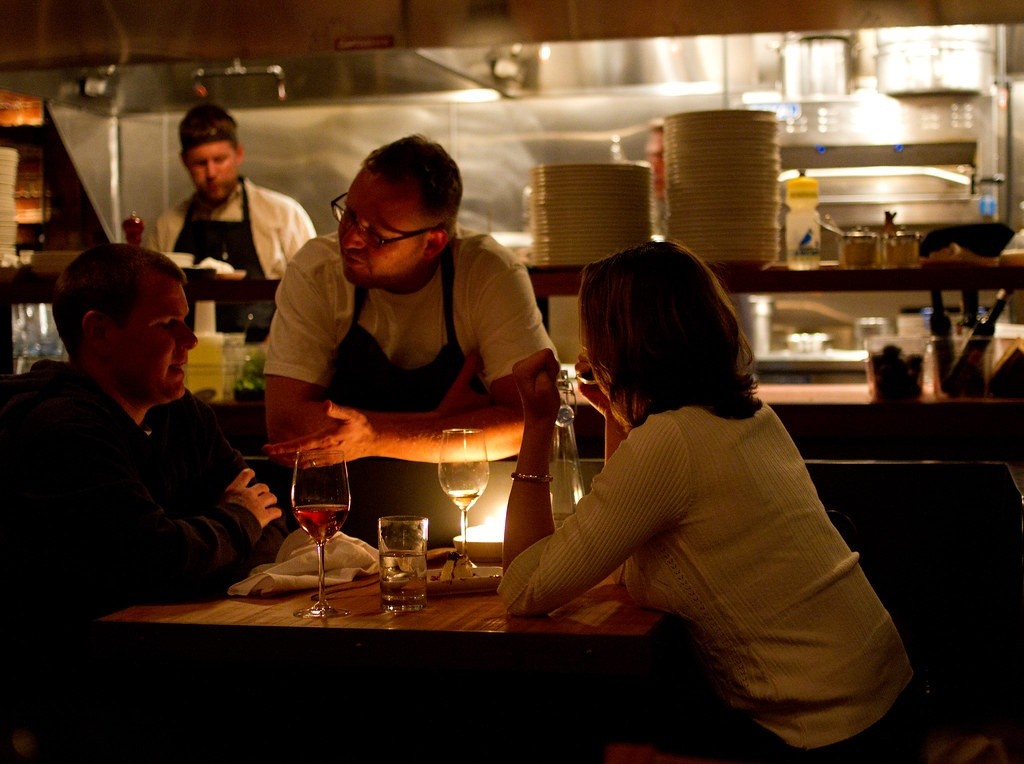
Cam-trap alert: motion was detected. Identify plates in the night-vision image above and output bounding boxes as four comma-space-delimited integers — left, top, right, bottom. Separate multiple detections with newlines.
0, 146, 21, 258
426, 566, 503, 597
662, 110, 782, 270
530, 161, 653, 264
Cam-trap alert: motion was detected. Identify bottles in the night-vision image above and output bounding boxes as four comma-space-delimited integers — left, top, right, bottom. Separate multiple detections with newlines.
646, 122, 664, 237
785, 169, 822, 271
927, 282, 1012, 399
547, 370, 586, 528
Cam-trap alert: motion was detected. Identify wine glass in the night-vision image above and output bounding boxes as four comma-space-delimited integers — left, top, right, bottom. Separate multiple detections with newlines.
438, 428, 490, 568
291, 449, 352, 618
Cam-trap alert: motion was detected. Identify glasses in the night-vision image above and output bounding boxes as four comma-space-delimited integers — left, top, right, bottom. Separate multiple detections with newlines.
331, 192, 450, 249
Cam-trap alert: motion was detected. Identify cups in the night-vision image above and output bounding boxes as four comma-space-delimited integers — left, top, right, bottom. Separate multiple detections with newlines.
864, 337, 927, 398
931, 336, 994, 397
736, 295, 776, 360
378, 515, 430, 614
12, 303, 66, 358
857, 315, 888, 349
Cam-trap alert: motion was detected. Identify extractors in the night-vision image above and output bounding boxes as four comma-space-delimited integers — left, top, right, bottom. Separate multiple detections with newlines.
777, 100, 976, 168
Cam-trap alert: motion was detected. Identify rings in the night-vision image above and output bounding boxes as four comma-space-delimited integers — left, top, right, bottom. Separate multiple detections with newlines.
312, 460, 316, 467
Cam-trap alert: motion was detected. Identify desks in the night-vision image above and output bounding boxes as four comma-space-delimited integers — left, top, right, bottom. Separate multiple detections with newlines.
91, 546, 672, 764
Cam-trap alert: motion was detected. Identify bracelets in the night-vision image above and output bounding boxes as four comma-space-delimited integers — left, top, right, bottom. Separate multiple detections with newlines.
509, 472, 552, 482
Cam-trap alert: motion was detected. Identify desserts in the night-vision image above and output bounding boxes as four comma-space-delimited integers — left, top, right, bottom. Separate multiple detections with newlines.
440, 551, 474, 581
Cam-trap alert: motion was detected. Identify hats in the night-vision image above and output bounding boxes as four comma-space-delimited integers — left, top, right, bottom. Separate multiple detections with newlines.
178, 103, 237, 148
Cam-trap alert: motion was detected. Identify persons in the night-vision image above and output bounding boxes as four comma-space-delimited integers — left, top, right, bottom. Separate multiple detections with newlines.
148, 104, 316, 342
0, 244, 286, 704
497, 240, 914, 764
263, 136, 559, 549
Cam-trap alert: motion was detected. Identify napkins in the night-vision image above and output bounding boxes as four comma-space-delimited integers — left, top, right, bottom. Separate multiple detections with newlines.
227, 527, 379, 597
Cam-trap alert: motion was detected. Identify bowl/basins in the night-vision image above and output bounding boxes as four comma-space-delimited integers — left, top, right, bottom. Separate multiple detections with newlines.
159, 251, 195, 266
453, 534, 503, 564
